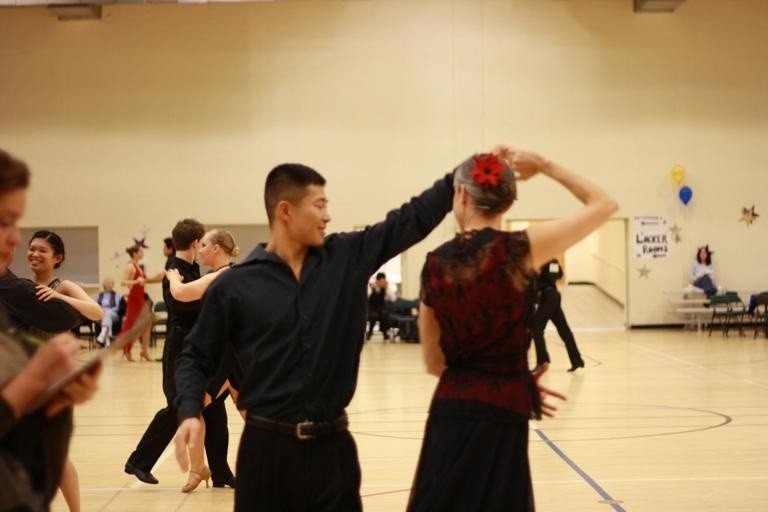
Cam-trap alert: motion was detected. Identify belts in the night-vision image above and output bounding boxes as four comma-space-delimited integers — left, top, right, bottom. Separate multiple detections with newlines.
245, 412, 349, 442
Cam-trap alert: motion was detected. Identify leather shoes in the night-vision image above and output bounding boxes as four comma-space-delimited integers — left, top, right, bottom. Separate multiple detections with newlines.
530, 360, 550, 374
568, 360, 585, 373
213, 477, 236, 490
124, 462, 160, 485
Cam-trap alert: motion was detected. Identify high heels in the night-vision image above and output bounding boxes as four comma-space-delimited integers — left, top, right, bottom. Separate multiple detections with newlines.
181, 466, 212, 493
122, 353, 135, 362
139, 352, 152, 362
177, 448, 190, 472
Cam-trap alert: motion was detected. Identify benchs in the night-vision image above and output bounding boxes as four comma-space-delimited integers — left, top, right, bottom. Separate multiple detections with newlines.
675, 308, 767, 333
667, 298, 750, 308
663, 287, 764, 299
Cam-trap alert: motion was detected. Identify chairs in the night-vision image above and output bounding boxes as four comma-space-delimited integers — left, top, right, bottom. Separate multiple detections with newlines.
708, 295, 746, 338
751, 291, 767, 337
736, 294, 767, 340
87, 321, 115, 350
152, 301, 169, 348
721, 291, 759, 337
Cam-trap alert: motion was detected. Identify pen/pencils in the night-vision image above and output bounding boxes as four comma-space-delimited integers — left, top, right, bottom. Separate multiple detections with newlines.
20, 333, 46, 347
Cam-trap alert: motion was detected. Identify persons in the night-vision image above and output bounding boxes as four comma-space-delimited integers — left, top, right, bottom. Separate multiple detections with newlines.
0, 266, 76, 335
406, 148, 620, 512
94, 277, 119, 348
530, 257, 584, 373
164, 228, 247, 494
125, 219, 239, 488
26, 230, 106, 512
690, 246, 717, 307
119, 285, 154, 348
140, 238, 176, 362
120, 242, 154, 362
366, 273, 396, 340
174, 145, 510, 512
0, 148, 99, 512
